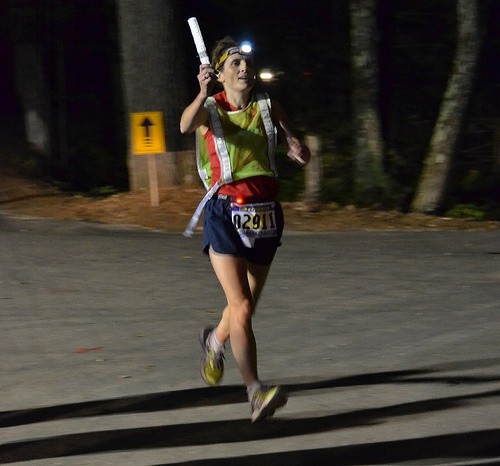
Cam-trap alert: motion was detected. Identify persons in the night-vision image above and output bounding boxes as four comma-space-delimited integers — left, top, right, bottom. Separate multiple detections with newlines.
180, 35, 312, 425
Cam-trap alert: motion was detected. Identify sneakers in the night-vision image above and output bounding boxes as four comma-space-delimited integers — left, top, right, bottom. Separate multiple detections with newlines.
199, 325, 227, 386
249, 381, 289, 425
